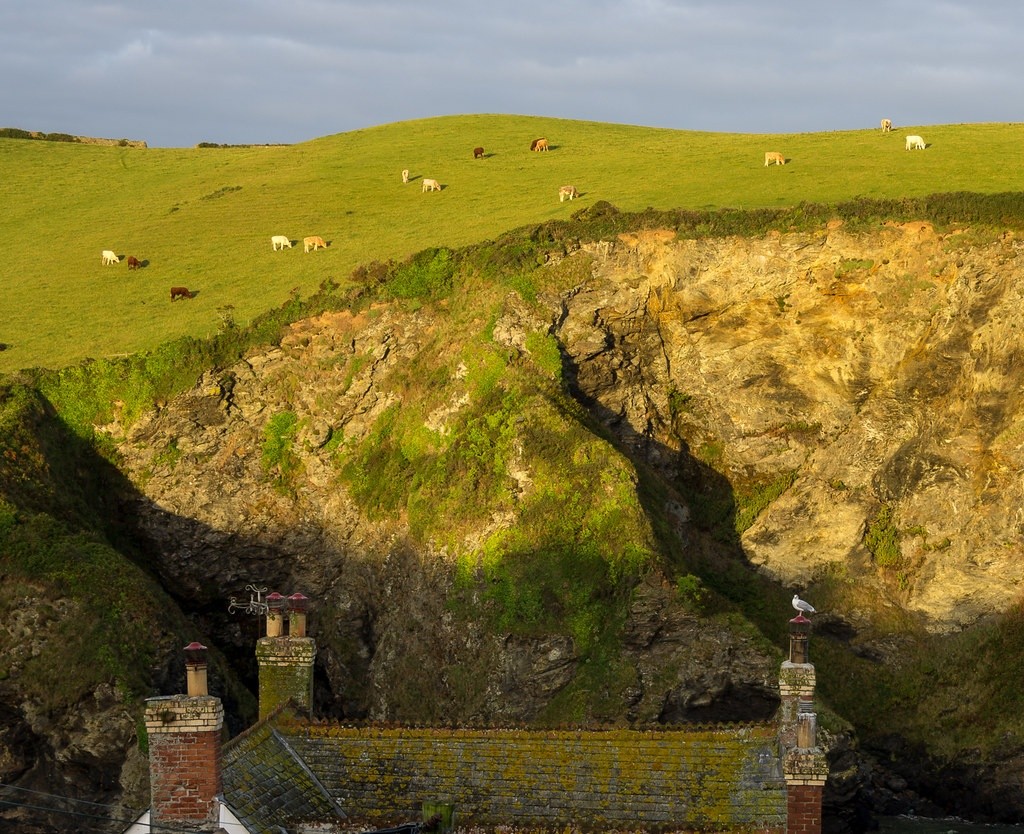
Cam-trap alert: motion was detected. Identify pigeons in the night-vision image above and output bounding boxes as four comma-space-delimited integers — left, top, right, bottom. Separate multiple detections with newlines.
792, 594, 818, 616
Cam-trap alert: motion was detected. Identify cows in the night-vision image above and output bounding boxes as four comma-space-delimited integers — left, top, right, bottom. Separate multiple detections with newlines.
473, 147, 484, 160
905, 135, 926, 150
102, 249, 120, 266
270, 236, 293, 251
764, 151, 785, 167
558, 186, 580, 202
529, 138, 548, 152
304, 236, 328, 253
402, 170, 409, 183
881, 119, 892, 133
171, 286, 193, 302
422, 178, 441, 193
128, 256, 142, 271
